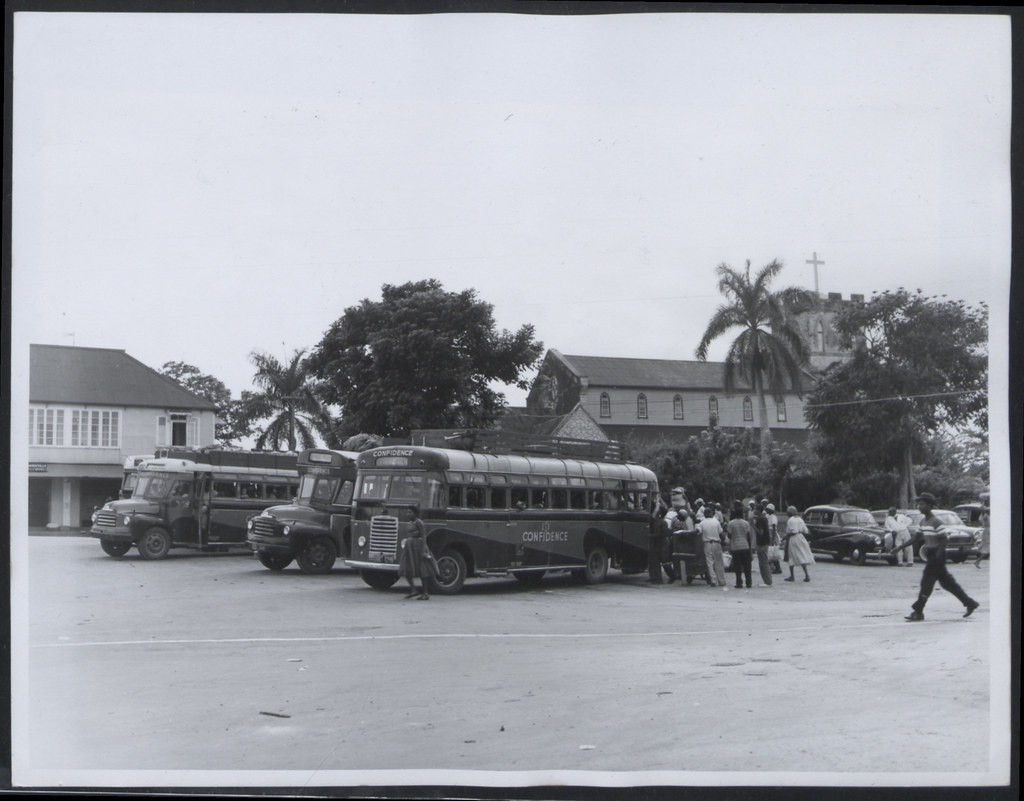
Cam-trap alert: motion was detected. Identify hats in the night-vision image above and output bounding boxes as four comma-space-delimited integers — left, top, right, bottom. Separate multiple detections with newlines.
766, 503, 775, 510
915, 492, 937, 505
694, 498, 705, 505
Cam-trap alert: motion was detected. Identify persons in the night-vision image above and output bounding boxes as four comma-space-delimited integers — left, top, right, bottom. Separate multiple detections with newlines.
398, 506, 439, 600
975, 497, 990, 569
890, 493, 979, 621
642, 487, 783, 588
200, 483, 297, 533
886, 508, 913, 567
781, 506, 815, 582
174, 487, 190, 506
152, 484, 166, 497
91, 496, 113, 523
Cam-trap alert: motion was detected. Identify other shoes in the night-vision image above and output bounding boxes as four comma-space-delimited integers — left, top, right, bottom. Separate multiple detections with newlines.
759, 583, 772, 587
898, 564, 902, 567
904, 611, 924, 620
907, 563, 912, 567
735, 585, 743, 588
975, 563, 981, 569
711, 583, 716, 587
771, 570, 782, 574
746, 585, 751, 588
804, 578, 809, 582
785, 578, 794, 581
963, 601, 979, 617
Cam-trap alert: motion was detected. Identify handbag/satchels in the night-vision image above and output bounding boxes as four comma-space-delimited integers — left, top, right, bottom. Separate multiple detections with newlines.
723, 551, 732, 567
767, 544, 781, 562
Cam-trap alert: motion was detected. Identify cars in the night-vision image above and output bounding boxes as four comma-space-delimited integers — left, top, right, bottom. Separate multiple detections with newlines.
797, 502, 990, 566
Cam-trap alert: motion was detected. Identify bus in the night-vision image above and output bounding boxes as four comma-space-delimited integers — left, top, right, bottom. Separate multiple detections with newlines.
89, 445, 304, 561
243, 447, 442, 575
340, 443, 664, 597
